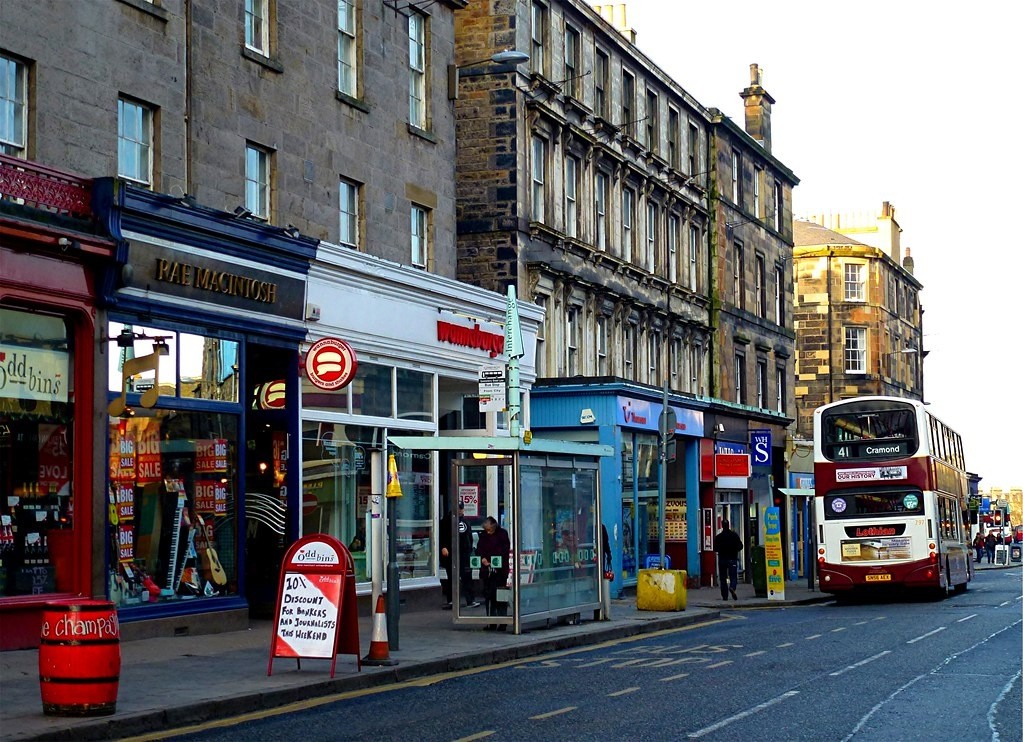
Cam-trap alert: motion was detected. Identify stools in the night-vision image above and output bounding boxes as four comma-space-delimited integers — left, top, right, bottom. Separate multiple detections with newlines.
38, 599, 121, 716
645, 554, 671, 571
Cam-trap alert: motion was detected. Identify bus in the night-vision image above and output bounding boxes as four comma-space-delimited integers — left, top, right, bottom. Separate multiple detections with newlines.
982, 502, 1011, 548
810, 393, 976, 601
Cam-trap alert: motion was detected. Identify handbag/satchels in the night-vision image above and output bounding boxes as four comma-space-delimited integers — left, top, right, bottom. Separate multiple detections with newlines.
602, 562, 614, 583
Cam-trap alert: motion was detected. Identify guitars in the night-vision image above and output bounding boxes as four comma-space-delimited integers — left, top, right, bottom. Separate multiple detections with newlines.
194, 511, 227, 586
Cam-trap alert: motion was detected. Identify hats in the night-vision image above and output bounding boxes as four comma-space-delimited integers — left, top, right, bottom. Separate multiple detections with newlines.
456, 501, 465, 509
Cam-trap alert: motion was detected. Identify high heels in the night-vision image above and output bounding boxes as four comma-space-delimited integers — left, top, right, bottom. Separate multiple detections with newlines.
604, 614, 610, 622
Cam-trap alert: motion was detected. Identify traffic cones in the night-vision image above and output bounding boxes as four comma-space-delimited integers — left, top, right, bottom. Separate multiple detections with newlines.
360, 593, 400, 667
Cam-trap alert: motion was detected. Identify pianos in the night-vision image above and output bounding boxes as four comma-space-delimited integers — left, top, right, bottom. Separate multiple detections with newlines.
152, 501, 197, 593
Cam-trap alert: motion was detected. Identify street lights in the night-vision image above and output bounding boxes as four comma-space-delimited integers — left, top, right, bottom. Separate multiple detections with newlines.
885, 347, 919, 396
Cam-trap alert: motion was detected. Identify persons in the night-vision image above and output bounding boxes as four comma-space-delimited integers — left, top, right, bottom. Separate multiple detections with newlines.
594, 522, 612, 620
973, 529, 1003, 565
350, 522, 366, 551
714, 520, 743, 600
441, 501, 508, 632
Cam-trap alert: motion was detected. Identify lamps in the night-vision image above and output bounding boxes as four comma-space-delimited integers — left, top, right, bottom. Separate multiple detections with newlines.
181, 193, 197, 208
888, 349, 917, 355
116, 328, 134, 348
152, 337, 169, 356
234, 206, 268, 223
59, 238, 80, 252
285, 223, 299, 237
448, 50, 530, 100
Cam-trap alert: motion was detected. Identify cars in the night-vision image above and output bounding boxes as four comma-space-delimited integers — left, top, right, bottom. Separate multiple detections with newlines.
1012, 525, 1023, 543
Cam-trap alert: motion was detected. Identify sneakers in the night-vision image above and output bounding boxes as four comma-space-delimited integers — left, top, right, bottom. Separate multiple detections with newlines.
447, 601, 453, 605
466, 601, 480, 608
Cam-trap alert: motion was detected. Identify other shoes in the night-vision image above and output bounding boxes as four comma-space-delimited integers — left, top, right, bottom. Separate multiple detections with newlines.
497, 624, 508, 631
729, 587, 737, 601
722, 595, 729, 601
483, 625, 497, 632
